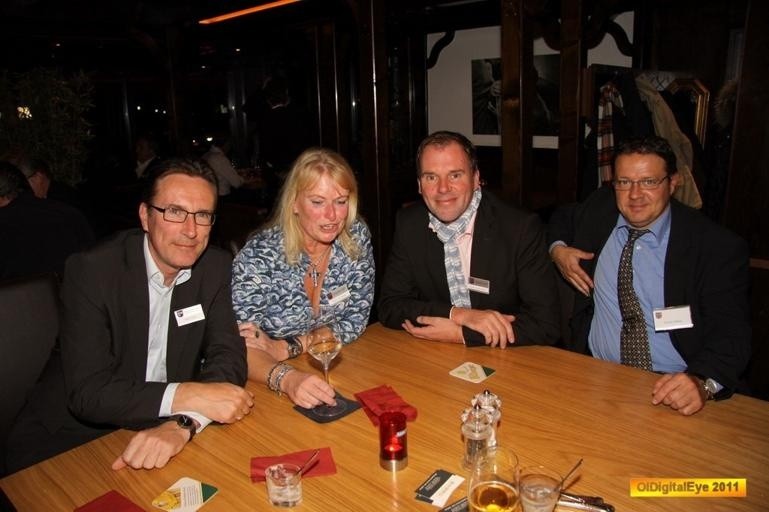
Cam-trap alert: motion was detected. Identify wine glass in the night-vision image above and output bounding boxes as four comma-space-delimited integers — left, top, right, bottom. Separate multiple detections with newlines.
306, 321, 346, 417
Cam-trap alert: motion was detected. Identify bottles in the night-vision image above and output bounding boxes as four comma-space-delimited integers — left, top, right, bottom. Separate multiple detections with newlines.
460, 385, 502, 471
376, 411, 411, 472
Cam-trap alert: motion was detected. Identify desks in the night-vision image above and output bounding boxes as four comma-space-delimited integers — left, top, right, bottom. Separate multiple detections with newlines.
1, 317, 769, 512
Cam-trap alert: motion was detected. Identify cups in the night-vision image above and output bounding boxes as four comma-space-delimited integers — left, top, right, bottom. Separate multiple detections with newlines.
265, 463, 304, 507
468, 443, 565, 511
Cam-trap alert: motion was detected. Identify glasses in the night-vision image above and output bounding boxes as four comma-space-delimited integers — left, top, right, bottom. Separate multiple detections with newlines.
612, 174, 669, 191
152, 205, 216, 226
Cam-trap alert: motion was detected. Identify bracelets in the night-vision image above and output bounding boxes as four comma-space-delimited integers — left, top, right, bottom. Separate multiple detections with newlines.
265, 362, 293, 395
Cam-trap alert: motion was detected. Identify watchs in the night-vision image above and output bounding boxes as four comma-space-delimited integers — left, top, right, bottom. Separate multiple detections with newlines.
165, 415, 198, 443
284, 335, 299, 359
697, 373, 717, 400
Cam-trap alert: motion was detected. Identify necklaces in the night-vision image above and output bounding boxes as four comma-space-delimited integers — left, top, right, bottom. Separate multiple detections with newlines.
302, 241, 329, 289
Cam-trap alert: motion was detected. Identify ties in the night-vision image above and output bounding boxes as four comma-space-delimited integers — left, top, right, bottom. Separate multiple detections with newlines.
616, 225, 654, 374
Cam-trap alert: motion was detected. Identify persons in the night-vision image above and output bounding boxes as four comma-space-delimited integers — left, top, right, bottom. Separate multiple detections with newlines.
1, 166, 93, 285
376, 131, 562, 350
547, 136, 749, 415
129, 137, 165, 186
204, 135, 258, 201
231, 147, 375, 412
5, 158, 255, 472
10, 155, 92, 209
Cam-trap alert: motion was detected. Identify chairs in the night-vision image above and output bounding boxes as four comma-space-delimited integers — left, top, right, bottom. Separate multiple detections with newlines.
0, 275, 64, 440
